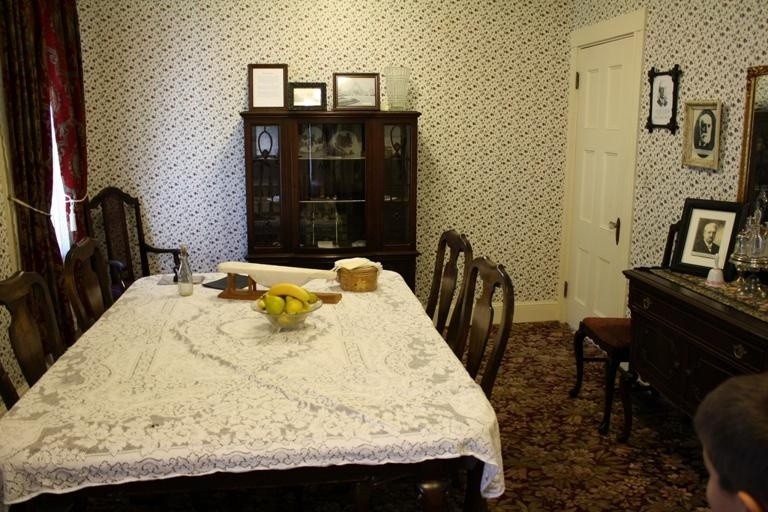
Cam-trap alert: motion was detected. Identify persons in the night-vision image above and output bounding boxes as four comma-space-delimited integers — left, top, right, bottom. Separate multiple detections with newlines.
695, 114, 713, 150
693, 223, 719, 254
657, 87, 668, 106
692, 373, 768, 512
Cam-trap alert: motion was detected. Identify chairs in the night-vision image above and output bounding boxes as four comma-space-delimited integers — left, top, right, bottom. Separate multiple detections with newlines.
62, 236, 127, 347
81, 186, 181, 303
0, 269, 68, 413
425, 229, 473, 362
453, 255, 514, 402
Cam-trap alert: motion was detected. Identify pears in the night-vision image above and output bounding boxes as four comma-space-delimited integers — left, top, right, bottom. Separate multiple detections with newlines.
258, 294, 303, 325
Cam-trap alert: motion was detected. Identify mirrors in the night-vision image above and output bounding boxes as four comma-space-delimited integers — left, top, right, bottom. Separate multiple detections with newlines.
735, 65, 768, 222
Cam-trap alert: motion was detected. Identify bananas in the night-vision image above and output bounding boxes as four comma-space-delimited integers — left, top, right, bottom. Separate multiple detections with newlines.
268, 283, 310, 304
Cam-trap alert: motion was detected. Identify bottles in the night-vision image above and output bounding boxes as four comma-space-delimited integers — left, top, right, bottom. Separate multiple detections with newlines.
176, 244, 194, 297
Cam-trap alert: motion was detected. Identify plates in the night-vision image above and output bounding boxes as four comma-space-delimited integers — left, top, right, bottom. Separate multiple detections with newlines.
298, 128, 358, 158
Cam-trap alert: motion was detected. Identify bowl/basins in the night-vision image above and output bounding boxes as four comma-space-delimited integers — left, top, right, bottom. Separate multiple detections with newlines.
250, 298, 323, 329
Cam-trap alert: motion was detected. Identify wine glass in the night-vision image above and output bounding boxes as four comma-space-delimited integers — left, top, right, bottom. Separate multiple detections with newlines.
729, 202, 768, 297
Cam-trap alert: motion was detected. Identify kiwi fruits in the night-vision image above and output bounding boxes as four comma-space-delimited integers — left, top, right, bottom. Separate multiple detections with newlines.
309, 294, 317, 304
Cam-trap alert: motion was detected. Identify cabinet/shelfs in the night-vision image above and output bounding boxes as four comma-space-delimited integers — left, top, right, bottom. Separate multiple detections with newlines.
238, 109, 421, 295
613, 265, 766, 466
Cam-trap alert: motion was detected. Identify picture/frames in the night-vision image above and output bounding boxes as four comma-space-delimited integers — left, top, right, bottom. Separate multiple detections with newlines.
247, 63, 289, 112
669, 197, 742, 282
287, 82, 327, 111
679, 98, 722, 173
332, 72, 381, 112
642, 63, 682, 137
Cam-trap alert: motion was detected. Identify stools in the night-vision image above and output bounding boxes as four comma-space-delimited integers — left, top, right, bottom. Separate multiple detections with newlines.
566, 317, 659, 436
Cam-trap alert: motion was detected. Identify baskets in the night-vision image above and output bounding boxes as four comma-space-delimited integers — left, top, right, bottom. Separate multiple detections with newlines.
338, 267, 379, 291
